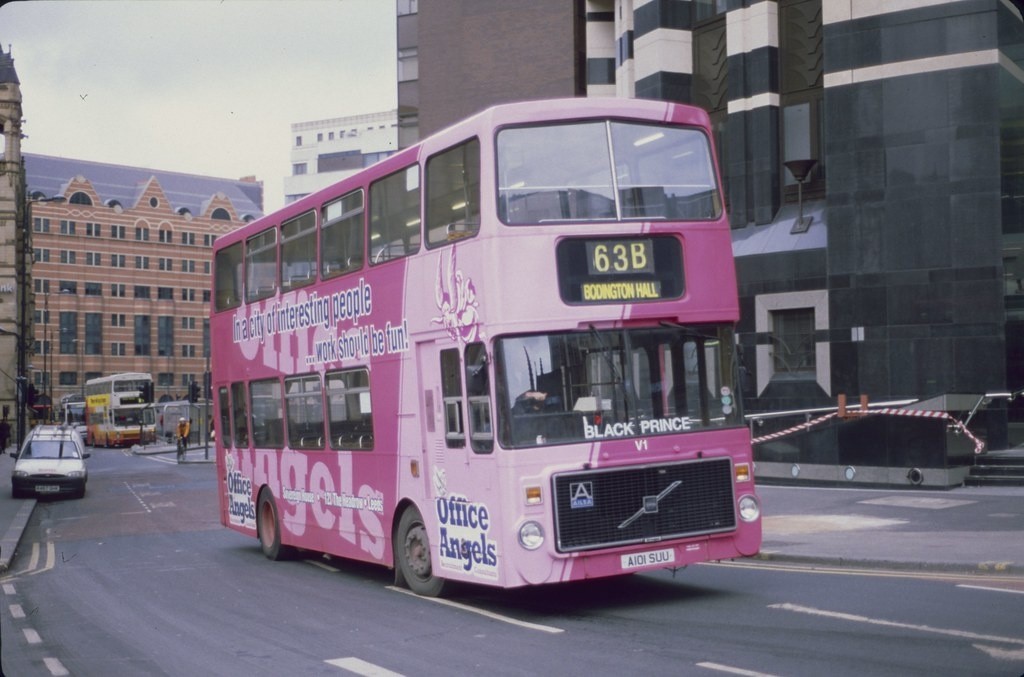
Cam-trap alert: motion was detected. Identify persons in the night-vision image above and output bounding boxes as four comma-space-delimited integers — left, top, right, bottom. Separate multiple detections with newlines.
208, 414, 214, 441
483, 361, 547, 429
176, 416, 190, 455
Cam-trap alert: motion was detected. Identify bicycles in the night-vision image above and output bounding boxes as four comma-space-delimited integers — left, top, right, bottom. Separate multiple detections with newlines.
174, 434, 187, 464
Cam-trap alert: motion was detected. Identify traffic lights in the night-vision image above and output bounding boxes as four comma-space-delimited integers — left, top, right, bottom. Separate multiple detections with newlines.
190, 381, 200, 403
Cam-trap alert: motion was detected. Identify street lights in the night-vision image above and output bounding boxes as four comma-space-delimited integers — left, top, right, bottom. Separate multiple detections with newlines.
17, 364, 34, 451
15, 194, 67, 448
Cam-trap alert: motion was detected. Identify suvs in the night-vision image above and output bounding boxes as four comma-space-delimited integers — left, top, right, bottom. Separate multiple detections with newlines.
9, 425, 90, 498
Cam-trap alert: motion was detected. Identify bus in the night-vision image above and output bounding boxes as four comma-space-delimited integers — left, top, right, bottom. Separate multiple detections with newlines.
61, 394, 85, 428
213, 93, 760, 599
85, 372, 156, 448
